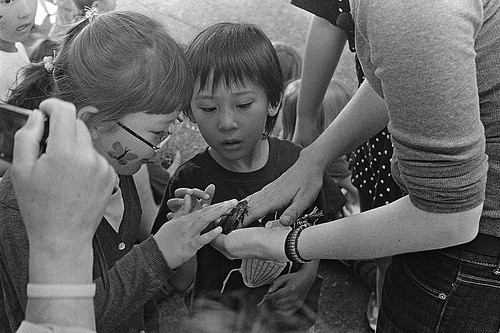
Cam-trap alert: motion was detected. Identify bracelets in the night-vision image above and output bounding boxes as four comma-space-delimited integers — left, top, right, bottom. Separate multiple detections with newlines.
285, 222, 313, 264
26, 282, 96, 298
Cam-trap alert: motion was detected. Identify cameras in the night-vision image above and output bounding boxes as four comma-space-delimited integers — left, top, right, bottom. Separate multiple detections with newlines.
0, 104, 52, 167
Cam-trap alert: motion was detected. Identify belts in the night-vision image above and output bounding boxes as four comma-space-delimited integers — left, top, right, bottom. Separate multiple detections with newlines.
458, 232, 500, 258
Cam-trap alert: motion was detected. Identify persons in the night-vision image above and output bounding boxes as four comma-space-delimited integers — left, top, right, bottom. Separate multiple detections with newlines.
1, 0, 500, 333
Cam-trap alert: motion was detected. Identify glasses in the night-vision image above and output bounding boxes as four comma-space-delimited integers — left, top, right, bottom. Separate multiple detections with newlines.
114, 116, 184, 153
